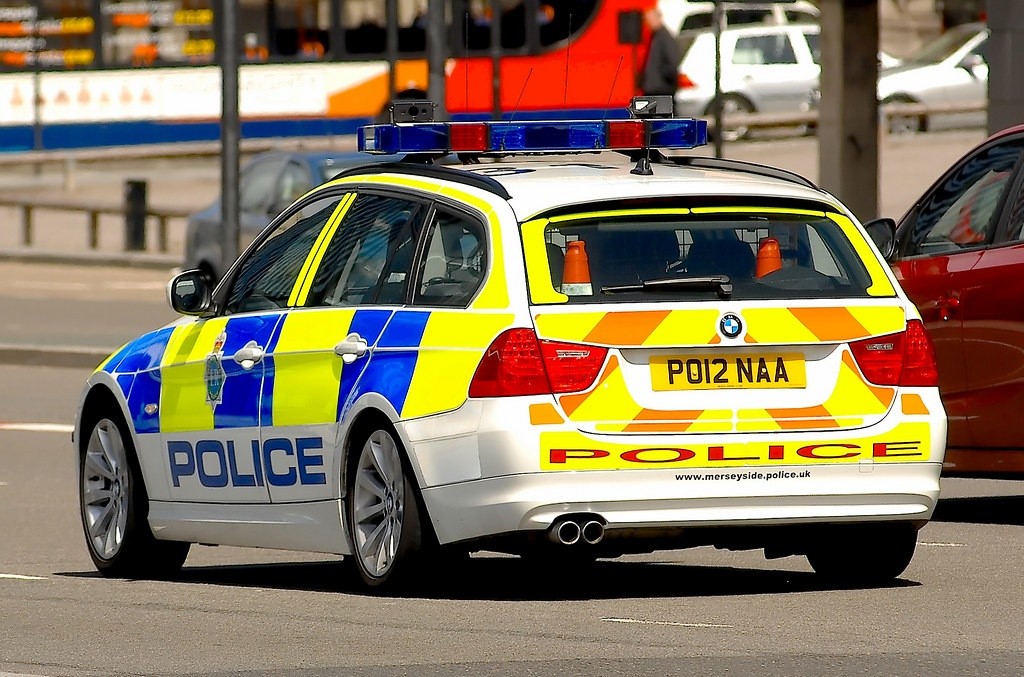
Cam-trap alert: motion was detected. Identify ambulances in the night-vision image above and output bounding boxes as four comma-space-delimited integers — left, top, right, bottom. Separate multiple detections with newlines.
71, 111, 949, 596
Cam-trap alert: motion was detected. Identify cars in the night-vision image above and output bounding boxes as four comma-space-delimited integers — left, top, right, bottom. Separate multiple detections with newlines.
864, 125, 1024, 481
874, 23, 991, 129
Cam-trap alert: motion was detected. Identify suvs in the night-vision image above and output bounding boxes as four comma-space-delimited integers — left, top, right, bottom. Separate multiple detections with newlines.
658, 0, 829, 60
671, 23, 899, 145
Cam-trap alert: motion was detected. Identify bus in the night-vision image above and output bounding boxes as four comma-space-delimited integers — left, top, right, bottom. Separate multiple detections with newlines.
0, 0, 672, 165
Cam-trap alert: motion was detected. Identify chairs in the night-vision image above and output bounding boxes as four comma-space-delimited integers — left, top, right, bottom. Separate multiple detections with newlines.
358, 215, 410, 307
687, 239, 754, 280
